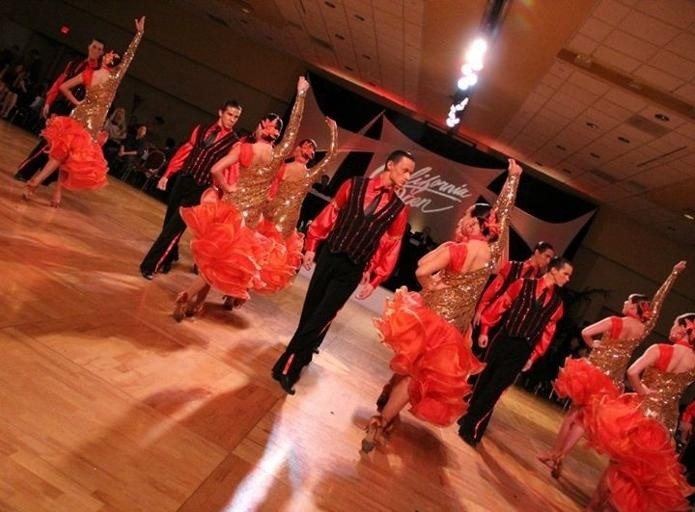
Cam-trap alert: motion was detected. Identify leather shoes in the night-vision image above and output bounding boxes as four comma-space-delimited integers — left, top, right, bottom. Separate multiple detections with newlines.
456, 414, 478, 448
360, 410, 398, 453
171, 289, 207, 323
270, 359, 298, 394
13, 170, 29, 181
535, 449, 565, 479
140, 249, 180, 280
21, 180, 66, 209
222, 291, 251, 310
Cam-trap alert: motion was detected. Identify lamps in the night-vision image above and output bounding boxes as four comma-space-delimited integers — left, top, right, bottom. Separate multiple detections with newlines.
445, 15, 503, 130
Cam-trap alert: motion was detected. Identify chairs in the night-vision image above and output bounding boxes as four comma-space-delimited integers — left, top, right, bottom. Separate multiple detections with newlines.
121, 148, 166, 192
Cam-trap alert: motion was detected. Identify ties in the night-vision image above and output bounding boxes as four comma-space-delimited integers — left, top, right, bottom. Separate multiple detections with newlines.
363, 186, 390, 221
74, 60, 89, 77
204, 124, 222, 149
535, 287, 550, 307
523, 265, 535, 276
